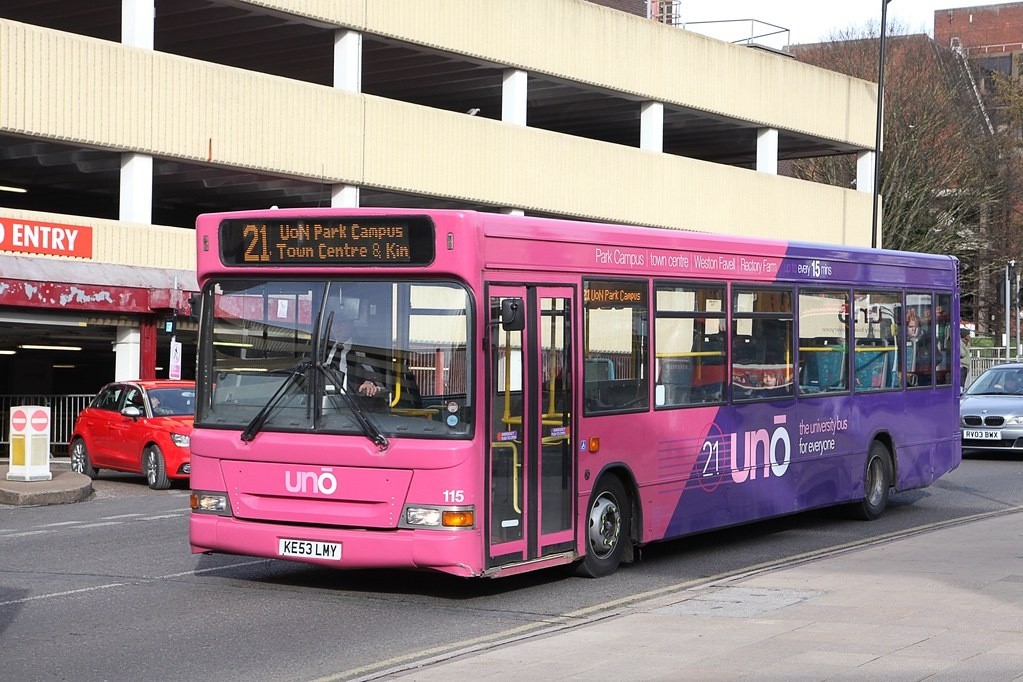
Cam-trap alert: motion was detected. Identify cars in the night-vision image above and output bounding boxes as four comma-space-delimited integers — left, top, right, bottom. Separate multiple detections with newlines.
960, 363, 1023, 450
69, 380, 214, 490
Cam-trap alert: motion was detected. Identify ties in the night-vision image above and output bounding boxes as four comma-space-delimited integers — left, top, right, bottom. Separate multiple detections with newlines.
330, 343, 344, 371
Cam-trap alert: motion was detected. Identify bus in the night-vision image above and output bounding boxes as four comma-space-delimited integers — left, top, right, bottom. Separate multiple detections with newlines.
187, 208, 963, 583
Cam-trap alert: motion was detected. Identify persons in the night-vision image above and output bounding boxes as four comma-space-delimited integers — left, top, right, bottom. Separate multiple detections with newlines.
149, 392, 173, 414
755, 370, 778, 387
293, 311, 386, 408
960, 328, 970, 398
906, 309, 943, 386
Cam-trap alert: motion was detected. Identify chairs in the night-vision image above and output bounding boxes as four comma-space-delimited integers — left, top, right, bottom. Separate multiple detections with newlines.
798, 335, 916, 392
577, 359, 614, 380
656, 332, 786, 404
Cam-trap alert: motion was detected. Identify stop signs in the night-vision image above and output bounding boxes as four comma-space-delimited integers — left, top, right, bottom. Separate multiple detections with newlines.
12, 410, 27, 432
30, 410, 48, 432
